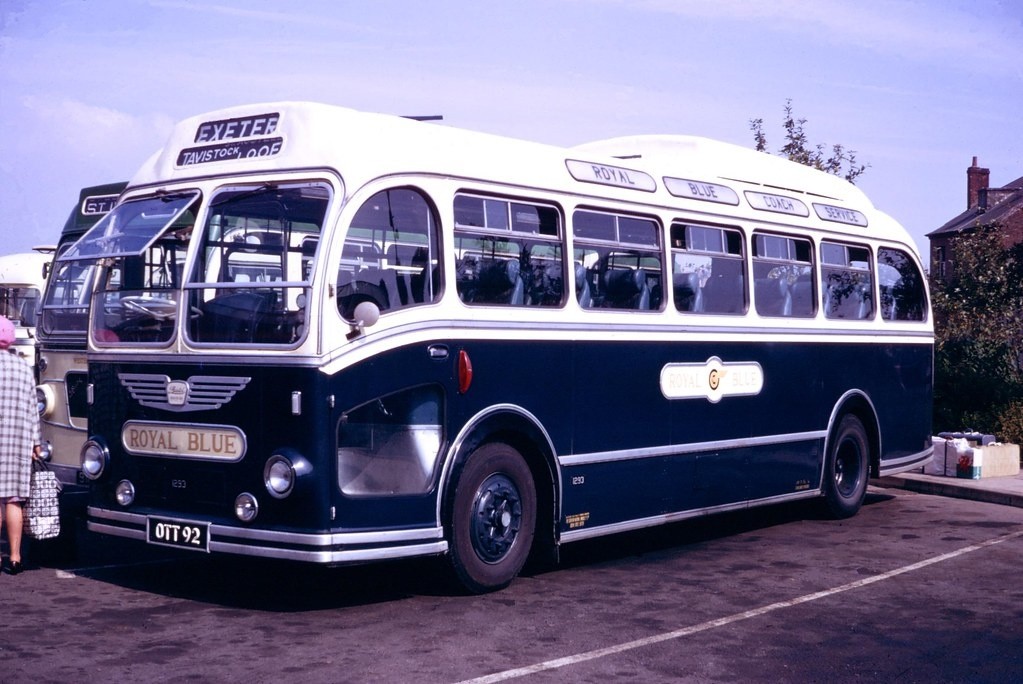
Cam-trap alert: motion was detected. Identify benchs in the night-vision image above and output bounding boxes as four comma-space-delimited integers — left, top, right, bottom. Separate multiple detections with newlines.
472, 260, 925, 321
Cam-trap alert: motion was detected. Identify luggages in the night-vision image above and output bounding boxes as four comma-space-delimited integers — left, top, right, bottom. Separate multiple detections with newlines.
923, 428, 1020, 479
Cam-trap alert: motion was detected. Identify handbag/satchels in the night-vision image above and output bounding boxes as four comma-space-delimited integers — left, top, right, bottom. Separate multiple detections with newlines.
21, 456, 63, 540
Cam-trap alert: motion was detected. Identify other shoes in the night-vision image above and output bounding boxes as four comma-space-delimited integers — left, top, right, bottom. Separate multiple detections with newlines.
12, 560, 25, 576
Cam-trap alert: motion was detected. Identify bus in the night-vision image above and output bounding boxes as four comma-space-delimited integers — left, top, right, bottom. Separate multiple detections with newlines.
0, 244, 425, 371
21, 181, 662, 489
55, 102, 935, 596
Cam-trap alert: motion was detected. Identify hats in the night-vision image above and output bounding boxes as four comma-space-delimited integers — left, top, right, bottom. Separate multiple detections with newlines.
0, 315, 16, 348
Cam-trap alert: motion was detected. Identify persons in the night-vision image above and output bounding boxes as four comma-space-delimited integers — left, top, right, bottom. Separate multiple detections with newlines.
0, 315, 42, 575
